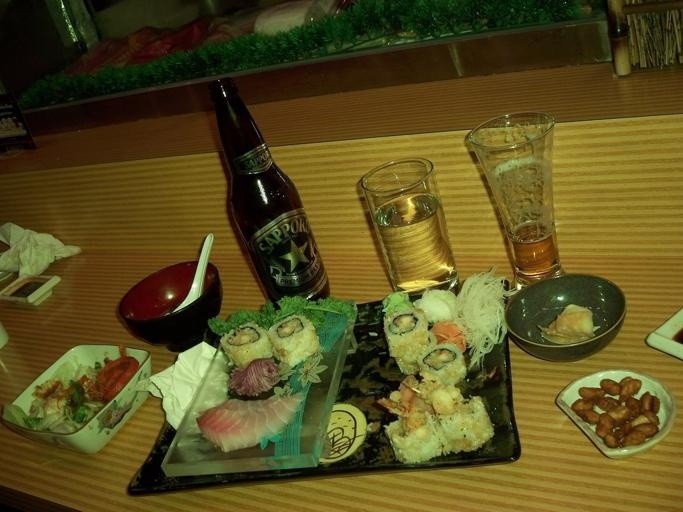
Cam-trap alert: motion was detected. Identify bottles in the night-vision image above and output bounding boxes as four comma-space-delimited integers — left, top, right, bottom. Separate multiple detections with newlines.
209, 76, 331, 308
50, 0, 100, 59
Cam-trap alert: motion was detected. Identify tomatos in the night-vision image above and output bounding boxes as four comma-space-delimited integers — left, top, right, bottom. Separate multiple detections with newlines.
98, 356, 138, 401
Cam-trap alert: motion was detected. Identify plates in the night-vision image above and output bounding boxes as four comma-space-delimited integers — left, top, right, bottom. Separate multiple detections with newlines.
554, 306, 683, 462
0, 273, 61, 307
125, 275, 521, 497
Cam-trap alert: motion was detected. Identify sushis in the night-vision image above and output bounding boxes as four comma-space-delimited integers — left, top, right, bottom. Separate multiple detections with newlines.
218, 323, 275, 370
382, 309, 496, 464
268, 314, 319, 366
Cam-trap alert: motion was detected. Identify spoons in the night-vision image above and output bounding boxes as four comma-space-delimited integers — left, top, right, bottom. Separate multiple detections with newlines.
163, 232, 213, 316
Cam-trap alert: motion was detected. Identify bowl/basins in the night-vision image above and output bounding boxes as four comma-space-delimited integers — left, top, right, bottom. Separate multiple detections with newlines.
2, 343, 152, 455
118, 262, 224, 350
507, 271, 627, 365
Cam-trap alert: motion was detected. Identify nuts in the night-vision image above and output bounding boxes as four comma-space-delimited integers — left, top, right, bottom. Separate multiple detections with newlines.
573, 376, 659, 448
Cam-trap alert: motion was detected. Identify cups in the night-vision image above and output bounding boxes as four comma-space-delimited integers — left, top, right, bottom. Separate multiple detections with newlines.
361, 112, 562, 302
